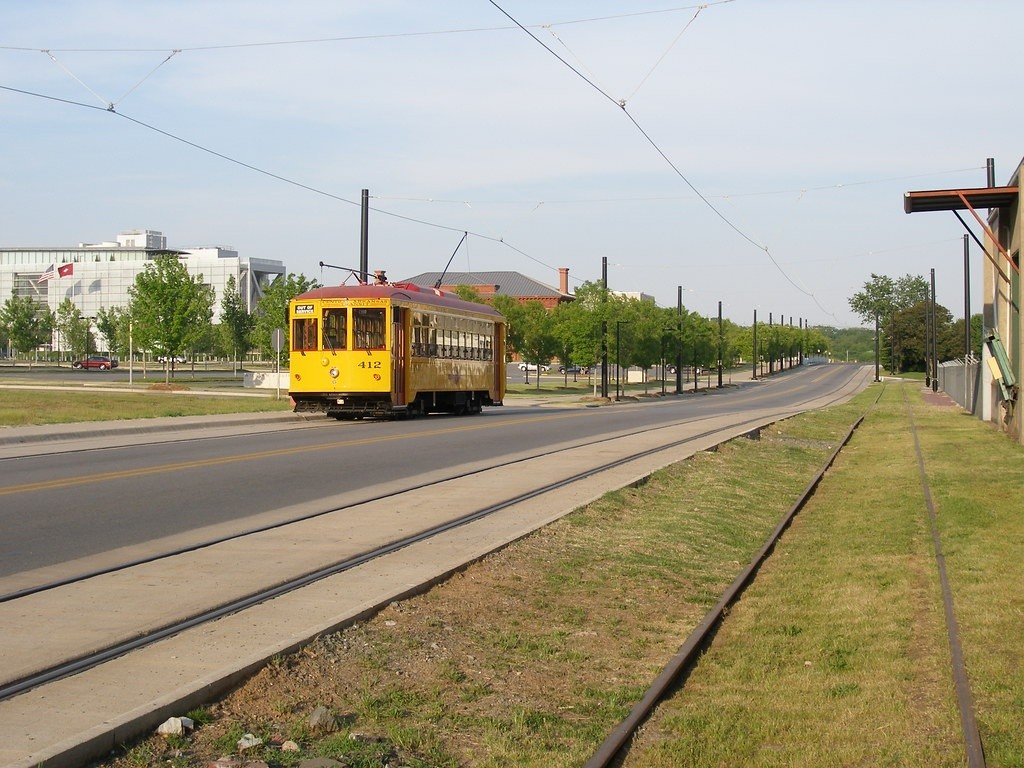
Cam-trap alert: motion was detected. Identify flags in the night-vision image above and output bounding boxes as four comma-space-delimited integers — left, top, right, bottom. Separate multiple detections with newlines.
58, 263, 73, 278
38, 265, 54, 283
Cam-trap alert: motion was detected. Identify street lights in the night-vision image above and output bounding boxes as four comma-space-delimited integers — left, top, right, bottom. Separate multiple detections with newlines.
659, 327, 677, 397
692, 334, 707, 394
614, 320, 629, 402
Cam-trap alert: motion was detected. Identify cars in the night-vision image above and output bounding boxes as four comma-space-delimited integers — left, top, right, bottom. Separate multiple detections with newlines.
664, 362, 708, 374
71, 355, 119, 371
157, 354, 187, 363
517, 361, 552, 372
557, 363, 589, 375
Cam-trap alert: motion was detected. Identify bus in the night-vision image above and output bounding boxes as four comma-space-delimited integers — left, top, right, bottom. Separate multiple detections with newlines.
283, 281, 509, 422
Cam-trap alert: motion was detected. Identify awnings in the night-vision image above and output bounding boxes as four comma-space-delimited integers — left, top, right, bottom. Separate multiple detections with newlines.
903, 186, 1020, 282
